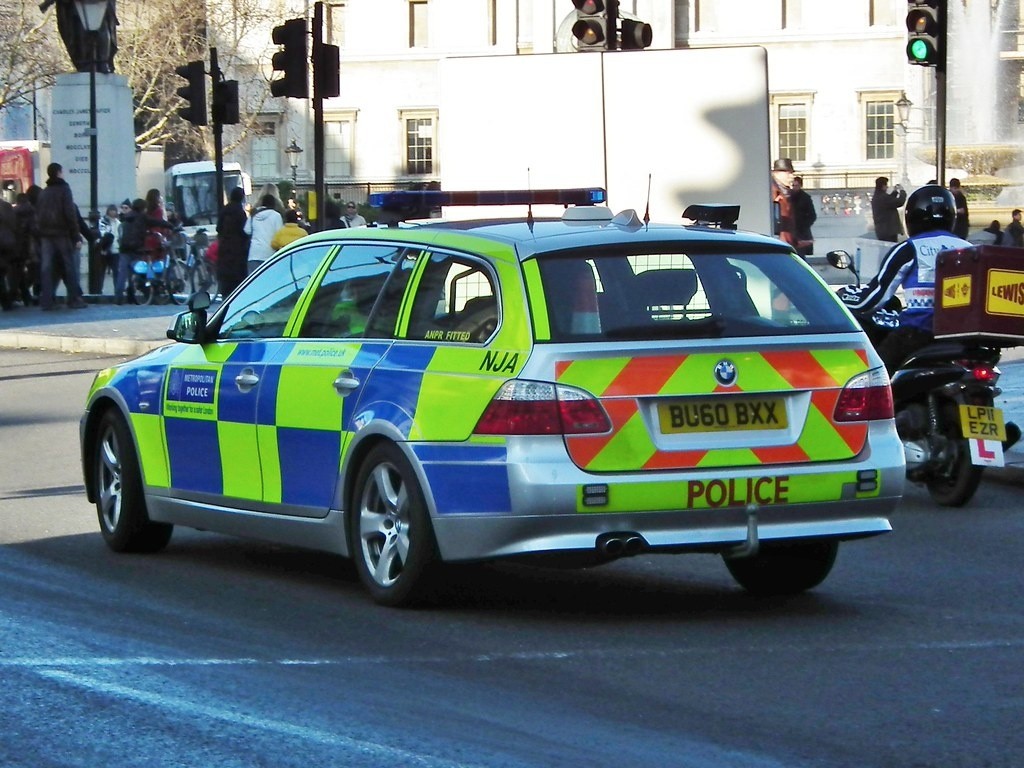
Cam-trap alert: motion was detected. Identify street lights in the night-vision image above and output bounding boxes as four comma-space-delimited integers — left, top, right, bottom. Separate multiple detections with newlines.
895, 94, 913, 185
285, 139, 305, 206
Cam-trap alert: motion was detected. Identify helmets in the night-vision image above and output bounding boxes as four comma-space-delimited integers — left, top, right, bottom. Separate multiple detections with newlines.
905, 185, 958, 235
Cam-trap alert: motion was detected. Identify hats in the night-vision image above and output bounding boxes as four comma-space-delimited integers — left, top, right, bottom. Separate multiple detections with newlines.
770, 158, 800, 173
122, 198, 131, 206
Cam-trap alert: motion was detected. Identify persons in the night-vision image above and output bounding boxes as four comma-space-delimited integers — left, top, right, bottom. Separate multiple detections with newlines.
254, 183, 367, 236
99, 188, 180, 306
773, 177, 816, 261
36, 163, 88, 311
0, 186, 101, 312
216, 188, 247, 299
837, 185, 975, 372
950, 179, 969, 240
872, 177, 907, 266
242, 195, 285, 277
1001, 209, 1024, 248
271, 210, 309, 251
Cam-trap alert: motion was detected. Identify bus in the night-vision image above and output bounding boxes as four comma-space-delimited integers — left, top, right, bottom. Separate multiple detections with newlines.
165, 161, 252, 243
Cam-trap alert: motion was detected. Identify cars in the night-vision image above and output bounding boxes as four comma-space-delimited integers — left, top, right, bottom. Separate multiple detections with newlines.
79, 188, 906, 610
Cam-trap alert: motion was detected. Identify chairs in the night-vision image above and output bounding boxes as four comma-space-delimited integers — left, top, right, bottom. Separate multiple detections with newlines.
624, 268, 699, 322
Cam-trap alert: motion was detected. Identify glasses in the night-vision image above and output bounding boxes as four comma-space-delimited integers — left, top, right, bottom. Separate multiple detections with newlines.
347, 205, 354, 209
120, 206, 129, 210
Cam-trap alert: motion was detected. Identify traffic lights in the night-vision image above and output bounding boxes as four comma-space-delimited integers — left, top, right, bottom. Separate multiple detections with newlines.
621, 20, 652, 50
176, 60, 207, 126
906, 0, 941, 65
572, 0, 608, 51
271, 18, 309, 99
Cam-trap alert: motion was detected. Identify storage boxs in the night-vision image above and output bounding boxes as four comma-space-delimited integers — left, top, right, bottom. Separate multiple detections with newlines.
933, 246, 1024, 348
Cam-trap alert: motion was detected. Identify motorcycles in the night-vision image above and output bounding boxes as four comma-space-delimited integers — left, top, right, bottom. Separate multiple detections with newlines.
824, 245, 1024, 508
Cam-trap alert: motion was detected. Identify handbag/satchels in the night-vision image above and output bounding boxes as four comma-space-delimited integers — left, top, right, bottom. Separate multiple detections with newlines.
101, 233, 114, 250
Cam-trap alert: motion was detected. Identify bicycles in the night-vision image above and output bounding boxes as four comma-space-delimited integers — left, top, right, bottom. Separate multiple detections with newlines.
129, 224, 218, 306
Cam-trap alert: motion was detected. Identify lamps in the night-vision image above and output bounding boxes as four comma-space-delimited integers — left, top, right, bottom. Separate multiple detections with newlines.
894, 91, 912, 123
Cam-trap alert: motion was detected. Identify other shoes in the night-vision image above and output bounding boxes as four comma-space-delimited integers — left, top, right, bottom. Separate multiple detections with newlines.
40, 299, 59, 311
68, 297, 89, 308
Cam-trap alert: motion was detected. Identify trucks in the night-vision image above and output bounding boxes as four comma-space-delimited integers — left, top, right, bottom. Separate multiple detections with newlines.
0, 141, 166, 218
